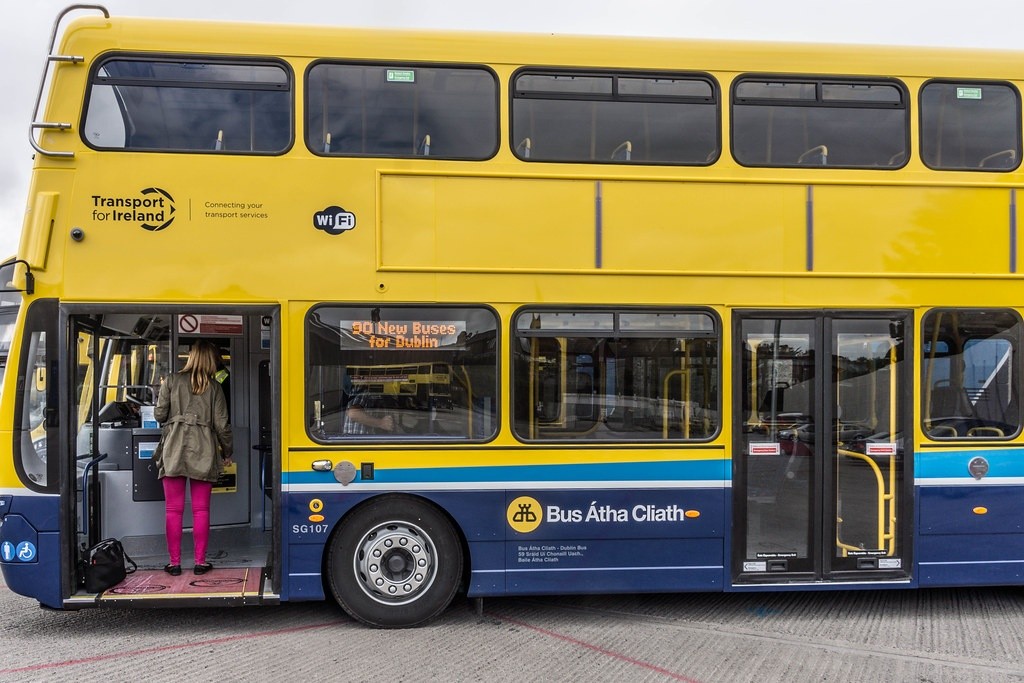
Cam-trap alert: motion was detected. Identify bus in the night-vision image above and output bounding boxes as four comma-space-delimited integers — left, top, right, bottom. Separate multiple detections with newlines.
345, 359, 454, 410
0, 0, 1024, 629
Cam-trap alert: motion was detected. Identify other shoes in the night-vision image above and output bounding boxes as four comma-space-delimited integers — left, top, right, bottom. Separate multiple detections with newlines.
194, 563, 213, 575
164, 564, 182, 576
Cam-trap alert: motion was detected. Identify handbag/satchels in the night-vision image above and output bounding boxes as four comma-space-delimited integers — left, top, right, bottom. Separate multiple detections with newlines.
82, 538, 137, 593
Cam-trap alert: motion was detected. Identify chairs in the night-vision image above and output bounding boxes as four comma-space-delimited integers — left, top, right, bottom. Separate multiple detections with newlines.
979, 148, 1016, 170
890, 151, 907, 165
215, 129, 225, 150
967, 426, 1005, 437
418, 135, 430, 156
798, 147, 828, 165
930, 426, 959, 437
934, 379, 976, 419
517, 138, 530, 158
324, 133, 331, 154
611, 141, 632, 161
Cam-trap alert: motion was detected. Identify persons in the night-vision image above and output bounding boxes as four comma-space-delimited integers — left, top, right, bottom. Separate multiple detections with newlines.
342, 388, 397, 435
154, 339, 233, 576
